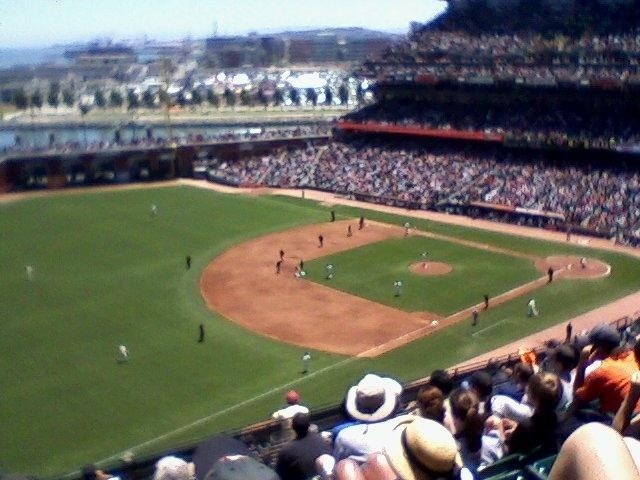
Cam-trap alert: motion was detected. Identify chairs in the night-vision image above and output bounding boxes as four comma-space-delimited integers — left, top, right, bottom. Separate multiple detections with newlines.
476, 439, 568, 480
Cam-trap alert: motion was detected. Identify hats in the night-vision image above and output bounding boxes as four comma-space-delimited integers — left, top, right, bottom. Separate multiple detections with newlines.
153, 436, 280, 480
286, 390, 300, 402
345, 373, 403, 423
381, 414, 463, 480
576, 324, 621, 352
